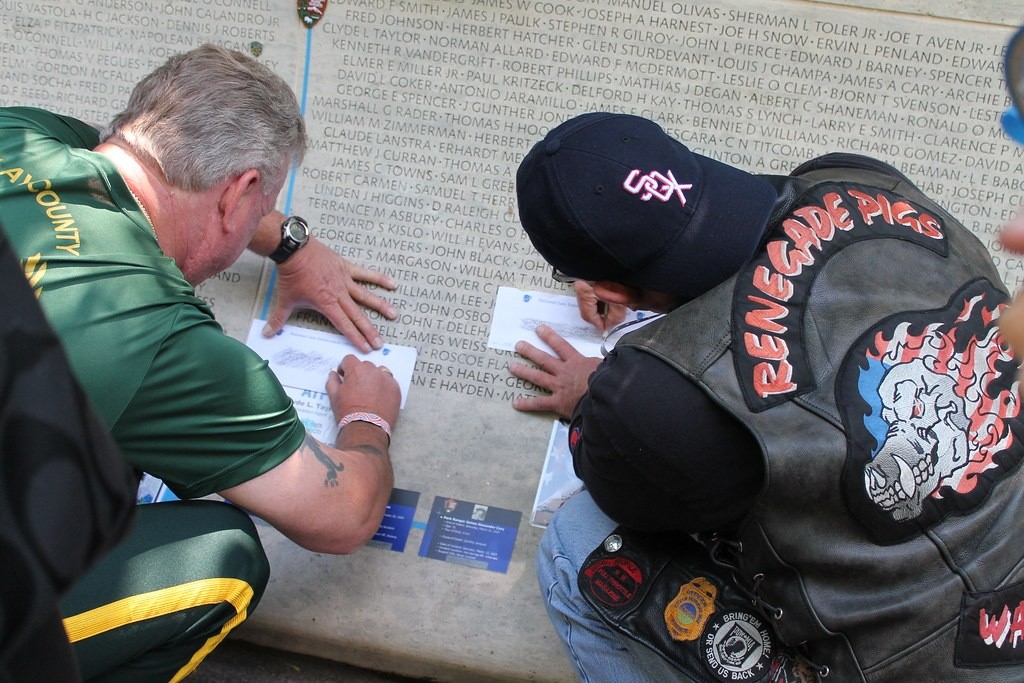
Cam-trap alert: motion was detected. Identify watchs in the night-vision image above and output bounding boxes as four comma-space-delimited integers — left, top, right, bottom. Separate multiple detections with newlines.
266, 215, 310, 264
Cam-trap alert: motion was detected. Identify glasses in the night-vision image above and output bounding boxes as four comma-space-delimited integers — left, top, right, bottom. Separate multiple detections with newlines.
551, 267, 596, 287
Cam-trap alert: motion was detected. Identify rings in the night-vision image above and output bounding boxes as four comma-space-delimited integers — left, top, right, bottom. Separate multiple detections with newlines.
380, 367, 394, 376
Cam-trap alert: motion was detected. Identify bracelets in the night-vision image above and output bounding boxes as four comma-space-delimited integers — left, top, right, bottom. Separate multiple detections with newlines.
336, 412, 394, 447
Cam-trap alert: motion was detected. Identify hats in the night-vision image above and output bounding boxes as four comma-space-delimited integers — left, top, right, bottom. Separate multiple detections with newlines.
515, 112, 777, 315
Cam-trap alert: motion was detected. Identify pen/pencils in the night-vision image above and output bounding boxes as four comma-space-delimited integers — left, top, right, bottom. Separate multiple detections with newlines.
598, 301, 608, 344
329, 367, 345, 384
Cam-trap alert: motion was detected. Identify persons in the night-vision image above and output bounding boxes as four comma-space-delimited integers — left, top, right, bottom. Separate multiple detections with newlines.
0, 45, 404, 683
505, 28, 1024, 683
